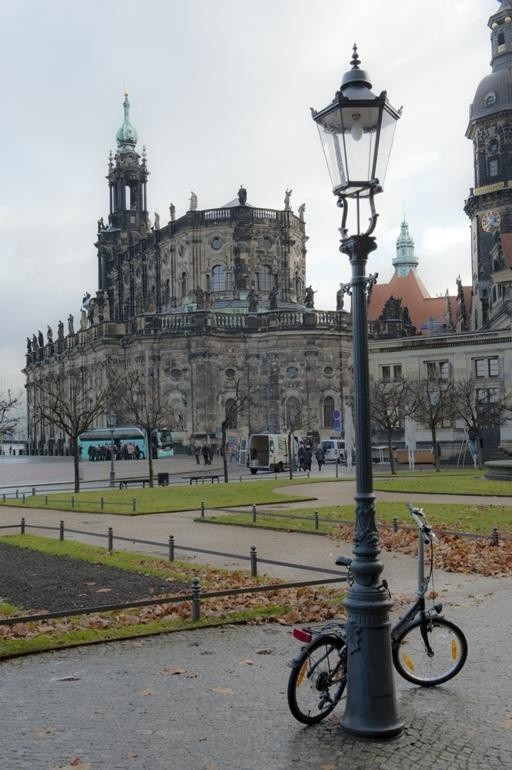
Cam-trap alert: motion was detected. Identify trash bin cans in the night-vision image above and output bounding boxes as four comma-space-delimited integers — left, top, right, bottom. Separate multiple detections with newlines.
158, 472, 169, 487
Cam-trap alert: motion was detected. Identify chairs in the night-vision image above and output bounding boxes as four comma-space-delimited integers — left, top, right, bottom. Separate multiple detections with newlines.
395, 448, 435, 470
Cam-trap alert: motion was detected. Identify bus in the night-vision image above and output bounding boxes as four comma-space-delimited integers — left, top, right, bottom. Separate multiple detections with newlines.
77, 427, 174, 460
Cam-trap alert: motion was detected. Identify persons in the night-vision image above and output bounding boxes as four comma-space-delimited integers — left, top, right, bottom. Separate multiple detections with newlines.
298, 203, 306, 221
26, 308, 89, 354
188, 189, 197, 210
336, 288, 345, 309
169, 203, 176, 220
195, 285, 205, 310
87, 442, 140, 462
236, 184, 248, 204
297, 444, 305, 471
154, 212, 161, 229
314, 444, 325, 470
194, 441, 240, 465
303, 445, 312, 471
283, 188, 293, 209
303, 285, 319, 305
268, 286, 279, 309
246, 285, 260, 312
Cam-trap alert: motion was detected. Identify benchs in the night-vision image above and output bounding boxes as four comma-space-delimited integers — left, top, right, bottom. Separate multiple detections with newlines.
108, 477, 150, 489
181, 474, 222, 484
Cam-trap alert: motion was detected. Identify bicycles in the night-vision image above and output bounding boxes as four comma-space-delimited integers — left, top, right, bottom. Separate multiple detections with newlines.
287, 500, 468, 725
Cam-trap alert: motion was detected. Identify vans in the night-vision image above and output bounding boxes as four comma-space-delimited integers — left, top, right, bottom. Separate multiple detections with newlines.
239, 433, 304, 474
320, 438, 355, 463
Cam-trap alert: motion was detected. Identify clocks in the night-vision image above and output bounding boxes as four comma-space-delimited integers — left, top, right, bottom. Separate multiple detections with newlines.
481, 209, 502, 233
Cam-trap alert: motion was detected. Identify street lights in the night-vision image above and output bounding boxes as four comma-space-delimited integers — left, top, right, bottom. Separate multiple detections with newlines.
308, 43, 406, 741
107, 410, 118, 487
